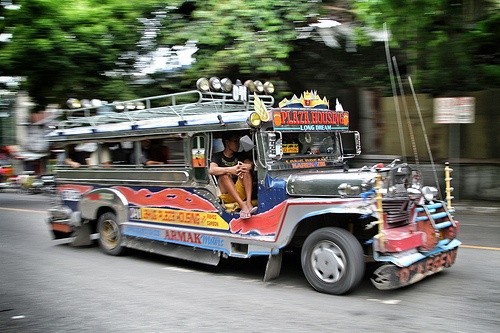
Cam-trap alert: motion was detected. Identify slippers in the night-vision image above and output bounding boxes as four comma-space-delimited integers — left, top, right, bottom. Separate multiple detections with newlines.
239, 210, 251, 219
250, 206, 258, 214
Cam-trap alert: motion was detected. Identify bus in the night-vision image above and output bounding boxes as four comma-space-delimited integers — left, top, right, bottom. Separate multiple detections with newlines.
46, 75, 463, 295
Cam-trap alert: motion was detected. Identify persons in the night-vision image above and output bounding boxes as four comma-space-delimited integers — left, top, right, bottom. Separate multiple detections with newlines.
90, 141, 119, 169
209, 131, 257, 219
64, 144, 81, 169
130, 139, 164, 166
